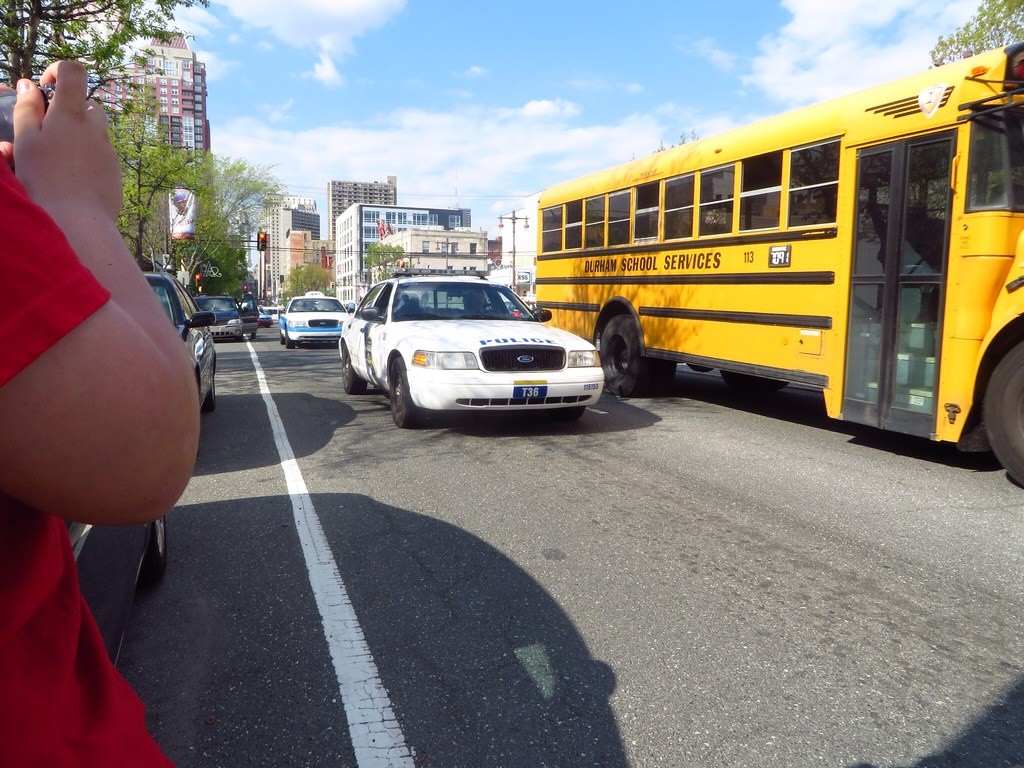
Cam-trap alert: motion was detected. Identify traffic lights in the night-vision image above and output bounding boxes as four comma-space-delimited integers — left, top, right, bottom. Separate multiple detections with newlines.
258, 232, 266, 251
244, 285, 247, 293
196, 275, 202, 287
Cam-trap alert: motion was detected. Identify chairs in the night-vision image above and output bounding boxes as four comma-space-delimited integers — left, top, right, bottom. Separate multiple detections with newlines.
393, 292, 425, 318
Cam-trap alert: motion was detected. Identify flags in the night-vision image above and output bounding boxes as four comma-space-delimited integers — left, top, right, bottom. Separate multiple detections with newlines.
170, 182, 196, 241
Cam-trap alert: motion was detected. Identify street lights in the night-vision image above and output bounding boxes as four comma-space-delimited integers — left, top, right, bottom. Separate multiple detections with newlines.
498, 210, 530, 291
435, 238, 459, 269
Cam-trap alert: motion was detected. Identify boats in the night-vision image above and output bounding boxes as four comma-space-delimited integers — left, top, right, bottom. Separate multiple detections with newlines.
338, 267, 606, 430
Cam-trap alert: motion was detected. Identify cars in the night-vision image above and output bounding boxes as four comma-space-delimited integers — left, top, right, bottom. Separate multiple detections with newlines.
143, 272, 217, 412
192, 294, 258, 342
278, 291, 355, 349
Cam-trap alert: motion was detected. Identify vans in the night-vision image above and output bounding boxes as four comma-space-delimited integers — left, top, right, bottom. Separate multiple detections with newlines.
236, 303, 273, 328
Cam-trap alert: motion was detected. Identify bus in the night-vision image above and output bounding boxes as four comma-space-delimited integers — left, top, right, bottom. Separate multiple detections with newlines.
261, 307, 284, 320
536, 40, 1024, 488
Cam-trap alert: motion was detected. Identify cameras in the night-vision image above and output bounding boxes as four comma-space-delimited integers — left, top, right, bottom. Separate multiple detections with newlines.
0, 82, 57, 143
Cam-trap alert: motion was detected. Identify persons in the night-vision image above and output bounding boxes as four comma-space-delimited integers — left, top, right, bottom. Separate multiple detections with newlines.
0, 60, 200, 768
